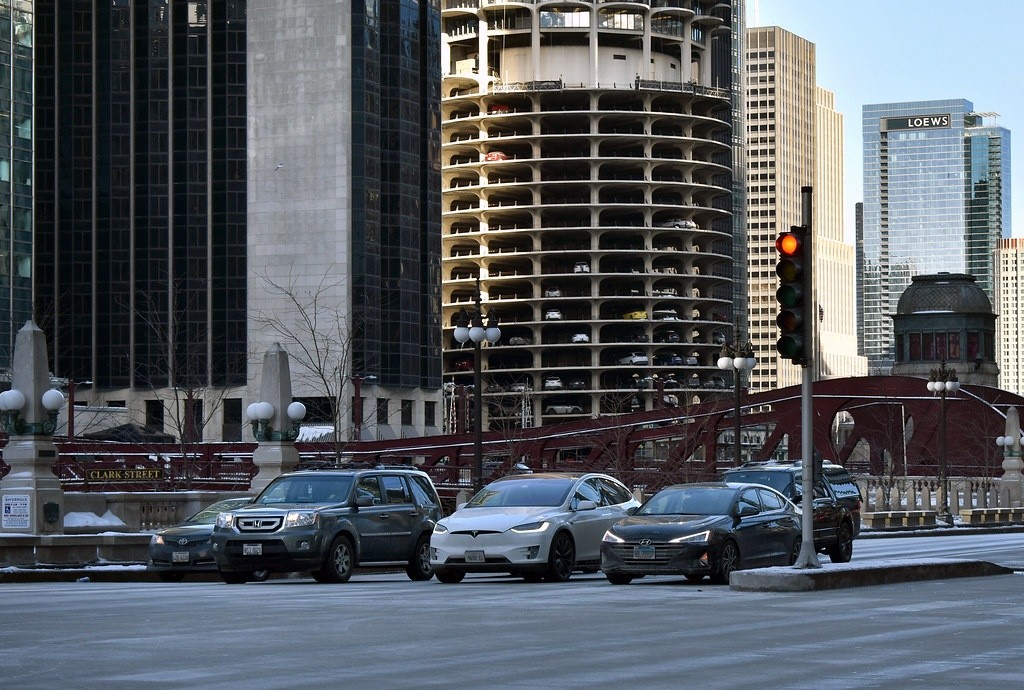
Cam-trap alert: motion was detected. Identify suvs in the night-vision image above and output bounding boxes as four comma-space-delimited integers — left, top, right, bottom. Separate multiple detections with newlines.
208, 462, 445, 585
719, 459, 865, 563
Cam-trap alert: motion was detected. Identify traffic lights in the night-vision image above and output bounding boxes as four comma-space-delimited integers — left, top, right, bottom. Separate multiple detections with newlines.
773, 229, 811, 363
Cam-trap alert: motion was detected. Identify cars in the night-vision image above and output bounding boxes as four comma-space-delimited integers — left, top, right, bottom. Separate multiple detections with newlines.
480, 461, 534, 475
428, 472, 650, 585
145, 496, 273, 582
483, 150, 505, 162
454, 218, 722, 413
597, 480, 805, 582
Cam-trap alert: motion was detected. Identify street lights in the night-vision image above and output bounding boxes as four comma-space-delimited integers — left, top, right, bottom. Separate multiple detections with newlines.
50, 378, 95, 439
927, 355, 964, 533
719, 327, 765, 467
448, 284, 506, 499
247, 343, 306, 499
989, 406, 1024, 509
0, 320, 75, 540
